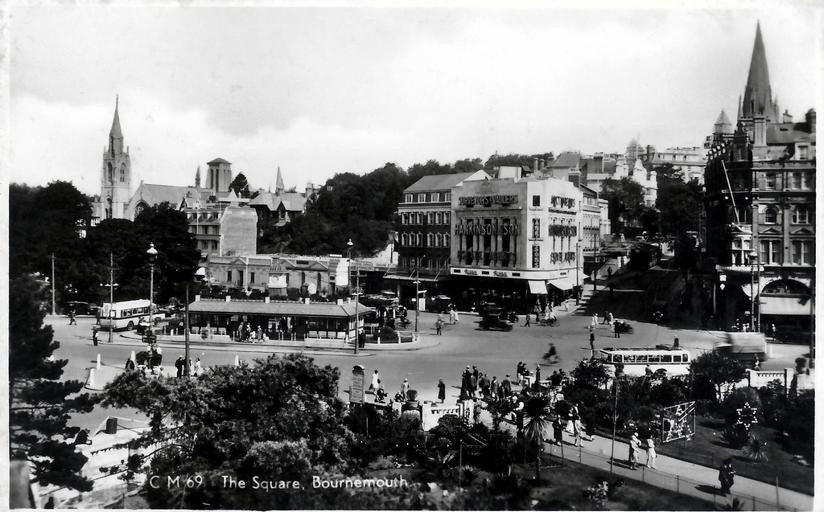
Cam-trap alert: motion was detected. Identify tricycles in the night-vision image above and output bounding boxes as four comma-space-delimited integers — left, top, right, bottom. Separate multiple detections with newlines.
713, 330, 768, 367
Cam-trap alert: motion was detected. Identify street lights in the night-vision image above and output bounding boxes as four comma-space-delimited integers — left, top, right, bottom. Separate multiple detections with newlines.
144, 240, 158, 332
748, 249, 758, 330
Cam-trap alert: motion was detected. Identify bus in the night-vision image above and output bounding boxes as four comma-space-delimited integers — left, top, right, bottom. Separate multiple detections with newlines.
98, 299, 157, 330
598, 344, 691, 381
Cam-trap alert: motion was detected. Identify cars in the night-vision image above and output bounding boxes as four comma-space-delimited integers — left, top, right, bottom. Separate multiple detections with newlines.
427, 294, 453, 313
478, 313, 513, 332
375, 302, 407, 318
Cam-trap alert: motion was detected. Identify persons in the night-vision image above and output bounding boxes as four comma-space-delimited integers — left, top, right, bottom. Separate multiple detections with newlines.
65, 299, 680, 471
